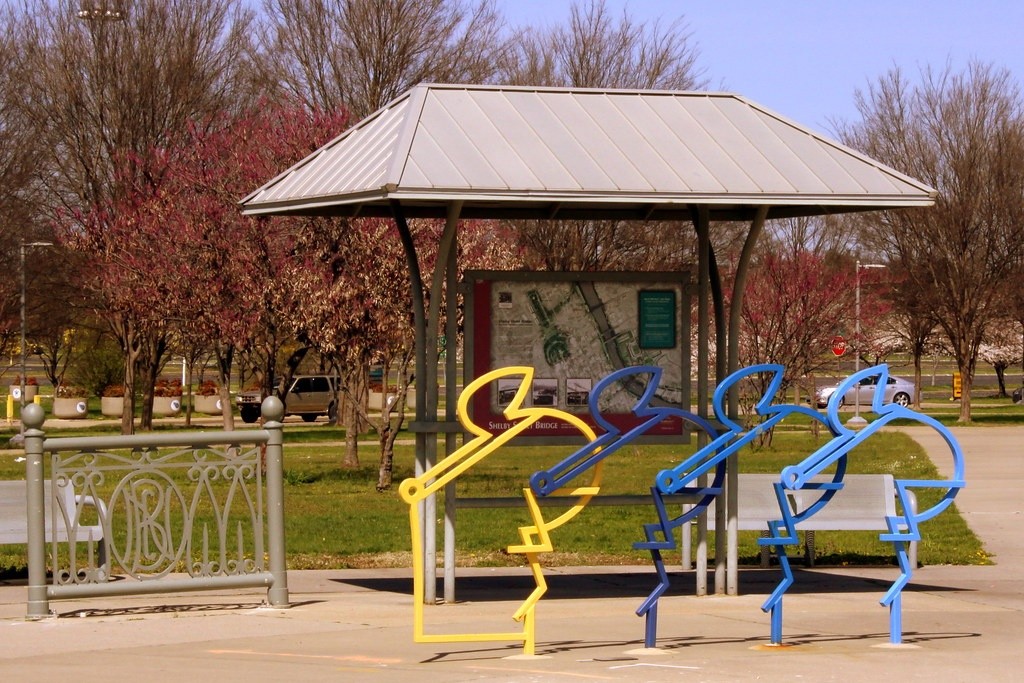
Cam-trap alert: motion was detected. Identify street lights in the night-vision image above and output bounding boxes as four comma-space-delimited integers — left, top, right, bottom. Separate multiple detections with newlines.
20, 242, 53, 435
856, 263, 885, 415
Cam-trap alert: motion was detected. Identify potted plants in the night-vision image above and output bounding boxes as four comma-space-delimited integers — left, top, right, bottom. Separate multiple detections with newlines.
368, 384, 397, 411
9, 374, 39, 402
194, 379, 223, 415
152, 378, 183, 416
406, 383, 417, 410
52, 386, 92, 418
101, 384, 125, 417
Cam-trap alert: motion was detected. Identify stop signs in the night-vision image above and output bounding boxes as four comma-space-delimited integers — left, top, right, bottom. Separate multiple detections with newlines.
830, 336, 847, 356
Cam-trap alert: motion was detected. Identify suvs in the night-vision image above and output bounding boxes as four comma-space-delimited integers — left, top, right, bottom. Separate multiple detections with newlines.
235, 375, 343, 424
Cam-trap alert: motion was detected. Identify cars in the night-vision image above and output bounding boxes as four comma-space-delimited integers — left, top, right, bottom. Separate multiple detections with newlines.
807, 374, 923, 411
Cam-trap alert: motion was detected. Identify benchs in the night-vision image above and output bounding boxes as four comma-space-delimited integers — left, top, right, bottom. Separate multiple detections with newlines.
0, 479, 106, 568
681, 472, 918, 569
